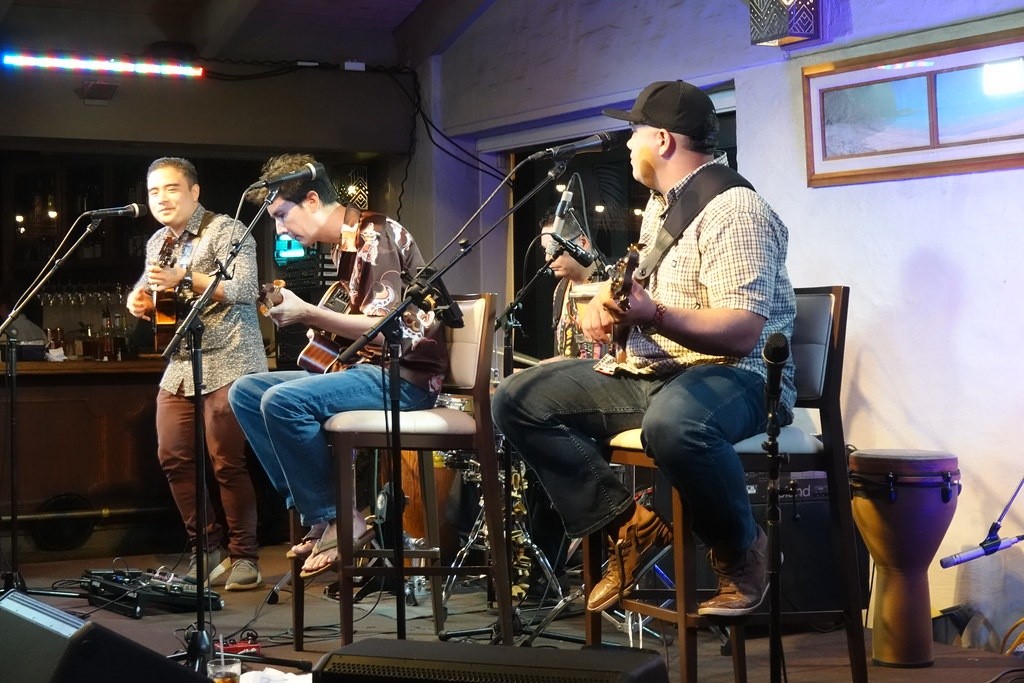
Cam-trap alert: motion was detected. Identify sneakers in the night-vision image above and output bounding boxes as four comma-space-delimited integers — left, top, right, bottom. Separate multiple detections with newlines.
588, 513, 673, 611
698, 526, 770, 616
184, 548, 221, 584
225, 558, 262, 589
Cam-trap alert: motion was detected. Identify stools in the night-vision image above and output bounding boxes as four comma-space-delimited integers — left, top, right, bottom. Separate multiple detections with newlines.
290, 285, 868, 683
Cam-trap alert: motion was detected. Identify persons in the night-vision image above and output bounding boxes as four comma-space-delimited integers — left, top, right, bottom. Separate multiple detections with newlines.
0, 305, 48, 342
244, 154, 449, 578
524, 210, 627, 598
492, 81, 797, 616
126, 157, 268, 590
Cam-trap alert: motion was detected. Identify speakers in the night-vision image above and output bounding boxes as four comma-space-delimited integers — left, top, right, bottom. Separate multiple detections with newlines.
0, 588, 216, 683
312, 637, 670, 683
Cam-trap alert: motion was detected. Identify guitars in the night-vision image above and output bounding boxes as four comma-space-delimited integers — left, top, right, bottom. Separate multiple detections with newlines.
254, 278, 352, 375
150, 236, 179, 354
609, 242, 640, 357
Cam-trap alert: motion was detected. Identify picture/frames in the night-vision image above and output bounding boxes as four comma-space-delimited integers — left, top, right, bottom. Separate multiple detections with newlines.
801, 27, 1024, 188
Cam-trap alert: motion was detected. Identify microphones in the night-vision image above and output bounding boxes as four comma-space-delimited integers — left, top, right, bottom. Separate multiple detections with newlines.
940, 535, 1024, 568
528, 130, 622, 162
84, 203, 148, 217
249, 162, 325, 190
546, 176, 575, 254
549, 232, 594, 268
761, 333, 790, 410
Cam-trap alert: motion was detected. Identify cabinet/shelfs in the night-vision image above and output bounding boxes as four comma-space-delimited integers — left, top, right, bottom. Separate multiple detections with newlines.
0, 150, 263, 295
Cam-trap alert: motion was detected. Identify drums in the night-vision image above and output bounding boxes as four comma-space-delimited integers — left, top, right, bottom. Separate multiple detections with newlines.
848, 449, 962, 668
379, 385, 495, 592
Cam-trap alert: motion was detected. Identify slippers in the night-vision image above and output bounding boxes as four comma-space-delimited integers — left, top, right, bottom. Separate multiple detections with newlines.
287, 526, 327, 559
299, 525, 374, 578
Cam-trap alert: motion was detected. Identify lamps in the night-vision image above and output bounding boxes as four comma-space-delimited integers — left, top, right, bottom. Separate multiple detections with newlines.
749, 0, 819, 47
331, 164, 367, 212
83, 80, 120, 105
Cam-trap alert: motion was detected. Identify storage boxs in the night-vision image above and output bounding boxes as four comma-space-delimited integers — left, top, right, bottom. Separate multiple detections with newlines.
1, 344, 45, 362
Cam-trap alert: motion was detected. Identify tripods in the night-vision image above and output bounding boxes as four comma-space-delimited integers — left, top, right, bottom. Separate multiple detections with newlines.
0, 216, 104, 599
159, 188, 313, 678
438, 245, 621, 646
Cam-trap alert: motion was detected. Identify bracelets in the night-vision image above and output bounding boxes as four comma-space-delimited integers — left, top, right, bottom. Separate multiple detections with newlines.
641, 302, 667, 335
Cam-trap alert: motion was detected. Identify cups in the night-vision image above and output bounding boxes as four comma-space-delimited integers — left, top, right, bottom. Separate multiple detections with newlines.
206, 658, 241, 683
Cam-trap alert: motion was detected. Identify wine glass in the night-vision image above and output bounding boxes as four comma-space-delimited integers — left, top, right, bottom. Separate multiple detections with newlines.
28, 277, 131, 306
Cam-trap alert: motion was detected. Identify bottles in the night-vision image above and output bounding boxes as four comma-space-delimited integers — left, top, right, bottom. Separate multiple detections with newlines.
79, 311, 131, 362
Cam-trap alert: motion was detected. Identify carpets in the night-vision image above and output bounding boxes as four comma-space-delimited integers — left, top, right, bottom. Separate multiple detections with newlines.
262, 567, 584, 622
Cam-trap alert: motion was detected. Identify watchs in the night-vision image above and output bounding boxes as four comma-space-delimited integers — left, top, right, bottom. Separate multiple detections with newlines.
182, 269, 191, 290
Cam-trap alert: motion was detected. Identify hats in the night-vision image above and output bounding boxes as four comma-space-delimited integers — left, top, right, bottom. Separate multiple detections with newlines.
603, 80, 720, 139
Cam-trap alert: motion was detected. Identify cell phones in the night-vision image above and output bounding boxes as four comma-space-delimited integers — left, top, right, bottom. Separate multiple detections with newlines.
417, 266, 463, 328
